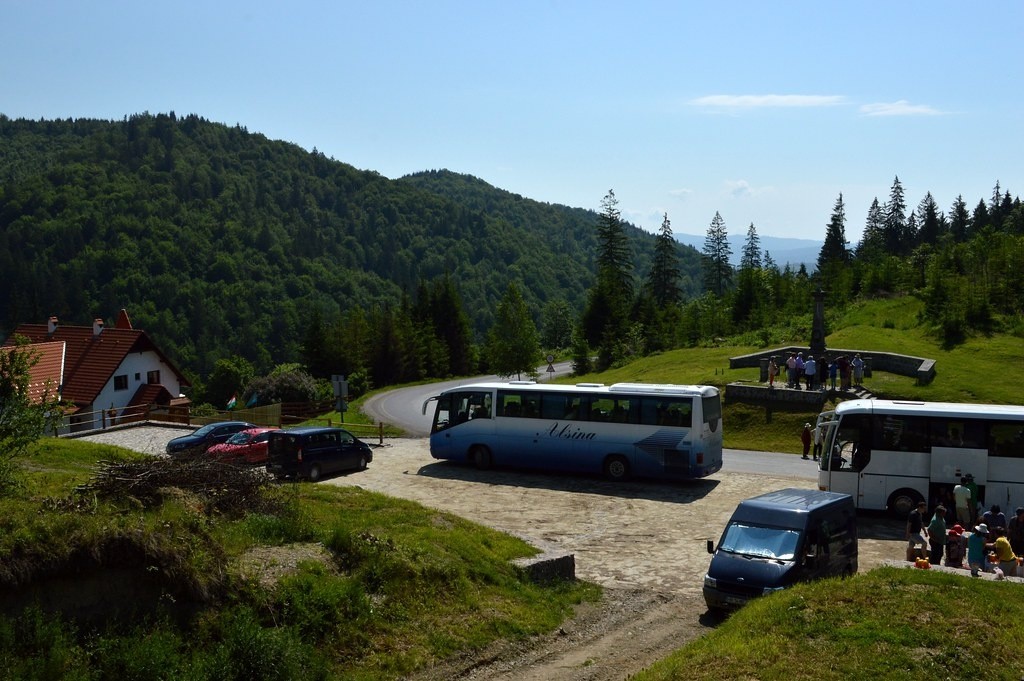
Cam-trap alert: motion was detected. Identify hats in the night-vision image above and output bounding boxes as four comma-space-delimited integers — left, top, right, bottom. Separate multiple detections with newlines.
805, 423, 811, 427
975, 523, 989, 533
799, 352, 803, 355
952, 524, 965, 533
948, 530, 960, 536
965, 473, 976, 480
792, 353, 796, 357
856, 353, 861, 357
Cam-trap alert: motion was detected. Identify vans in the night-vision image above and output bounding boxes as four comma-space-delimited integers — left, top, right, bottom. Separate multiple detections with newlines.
703, 488, 857, 615
266, 430, 374, 478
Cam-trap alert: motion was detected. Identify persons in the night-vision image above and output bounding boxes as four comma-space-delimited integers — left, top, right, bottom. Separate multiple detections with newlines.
829, 361, 838, 390
944, 524, 967, 568
986, 526, 1018, 576
801, 422, 811, 459
851, 353, 863, 387
810, 426, 822, 461
768, 356, 778, 387
796, 352, 808, 389
952, 473, 1024, 559
803, 355, 816, 391
786, 352, 797, 388
906, 502, 929, 560
927, 504, 947, 565
815, 356, 830, 390
969, 523, 996, 571
471, 402, 488, 419
839, 355, 850, 391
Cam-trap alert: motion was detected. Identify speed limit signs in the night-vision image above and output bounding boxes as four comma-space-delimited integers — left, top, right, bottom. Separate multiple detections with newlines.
547, 354, 554, 363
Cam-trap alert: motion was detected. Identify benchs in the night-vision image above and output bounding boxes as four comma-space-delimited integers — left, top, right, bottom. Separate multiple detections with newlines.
506, 400, 518, 417
593, 407, 602, 421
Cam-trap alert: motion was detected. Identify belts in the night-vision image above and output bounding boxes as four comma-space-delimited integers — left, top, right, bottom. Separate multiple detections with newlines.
1001, 558, 1017, 562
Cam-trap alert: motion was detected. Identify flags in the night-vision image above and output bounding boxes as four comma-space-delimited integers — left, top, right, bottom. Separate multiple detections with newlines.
246, 393, 257, 407
226, 394, 236, 410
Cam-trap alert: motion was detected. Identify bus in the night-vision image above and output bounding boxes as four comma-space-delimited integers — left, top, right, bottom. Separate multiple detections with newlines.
813, 399, 1024, 528
413, 381, 722, 486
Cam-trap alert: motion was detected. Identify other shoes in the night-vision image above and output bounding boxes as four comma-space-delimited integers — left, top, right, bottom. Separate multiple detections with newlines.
769, 385, 861, 391
803, 456, 809, 459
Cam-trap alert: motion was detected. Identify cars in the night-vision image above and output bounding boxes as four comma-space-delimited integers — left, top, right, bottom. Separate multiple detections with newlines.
164, 422, 258, 458
205, 427, 285, 468
